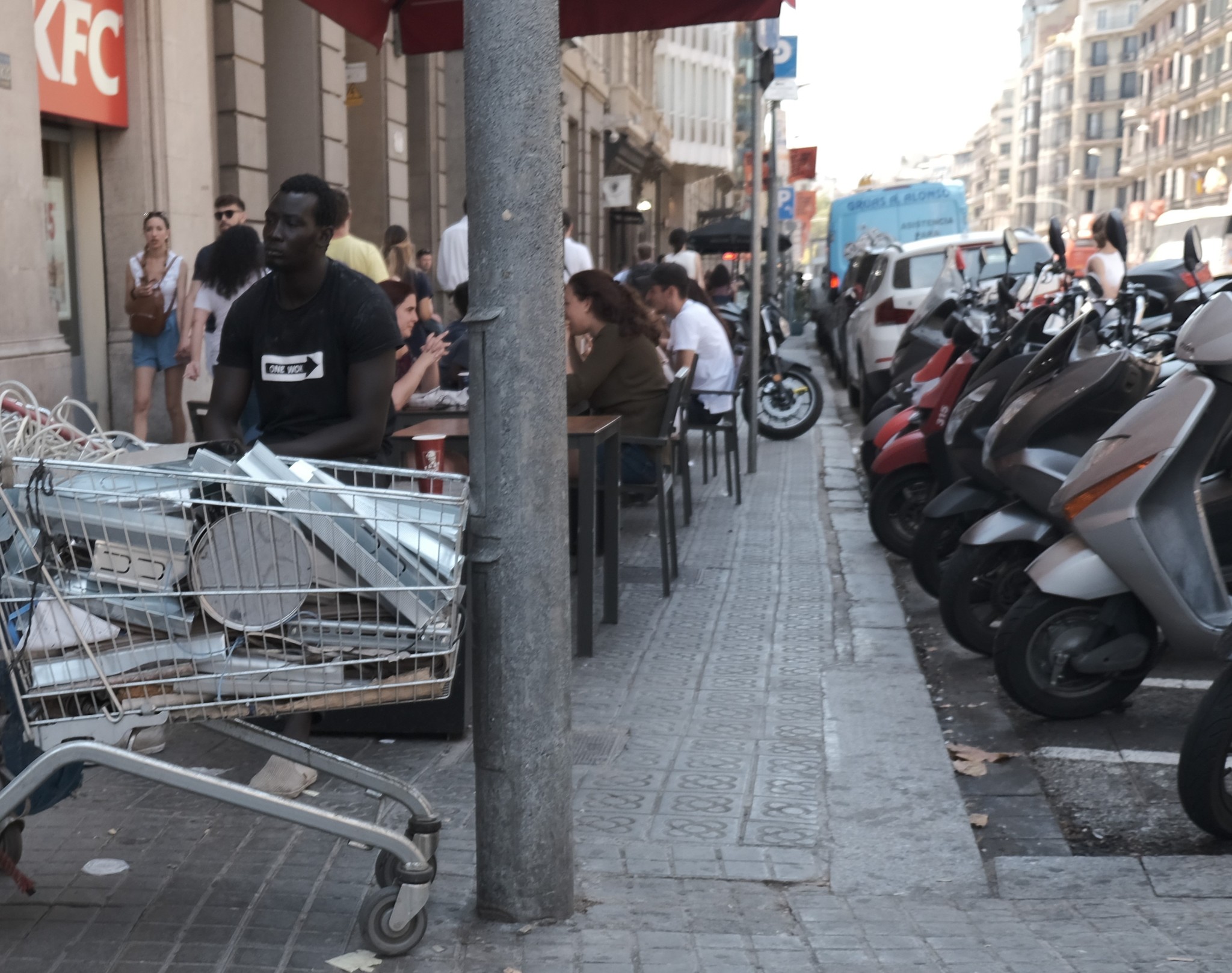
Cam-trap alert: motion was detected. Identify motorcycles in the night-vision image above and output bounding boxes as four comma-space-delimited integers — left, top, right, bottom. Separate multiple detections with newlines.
712, 289, 825, 443
707, 284, 827, 442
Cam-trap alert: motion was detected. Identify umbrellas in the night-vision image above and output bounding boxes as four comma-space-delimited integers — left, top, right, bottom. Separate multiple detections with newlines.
685, 213, 792, 290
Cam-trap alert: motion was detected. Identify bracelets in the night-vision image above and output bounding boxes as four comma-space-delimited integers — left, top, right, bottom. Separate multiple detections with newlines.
130, 287, 137, 300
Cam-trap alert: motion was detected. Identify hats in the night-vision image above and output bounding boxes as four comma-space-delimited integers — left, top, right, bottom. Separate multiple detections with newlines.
634, 261, 690, 285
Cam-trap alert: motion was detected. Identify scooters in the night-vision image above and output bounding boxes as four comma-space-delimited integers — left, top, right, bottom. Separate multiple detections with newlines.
859, 203, 1232, 844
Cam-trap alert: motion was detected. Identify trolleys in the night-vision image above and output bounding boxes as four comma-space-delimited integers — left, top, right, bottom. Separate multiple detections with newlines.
0, 394, 472, 959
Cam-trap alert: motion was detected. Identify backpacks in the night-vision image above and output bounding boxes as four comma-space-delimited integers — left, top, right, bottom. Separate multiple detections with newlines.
129, 251, 178, 337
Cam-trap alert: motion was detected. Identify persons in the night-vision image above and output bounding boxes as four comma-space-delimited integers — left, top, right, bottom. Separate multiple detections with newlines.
1086, 211, 1124, 301
560, 206, 750, 578
122, 211, 190, 445
202, 172, 402, 487
174, 195, 270, 451
326, 190, 468, 496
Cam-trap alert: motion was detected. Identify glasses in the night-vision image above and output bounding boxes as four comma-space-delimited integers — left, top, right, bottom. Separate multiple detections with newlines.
214, 210, 242, 221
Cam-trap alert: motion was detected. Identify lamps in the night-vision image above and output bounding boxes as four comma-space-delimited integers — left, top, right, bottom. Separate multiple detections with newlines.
602, 126, 620, 144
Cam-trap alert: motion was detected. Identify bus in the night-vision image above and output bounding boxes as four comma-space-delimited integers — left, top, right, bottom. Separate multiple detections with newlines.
820, 179, 968, 336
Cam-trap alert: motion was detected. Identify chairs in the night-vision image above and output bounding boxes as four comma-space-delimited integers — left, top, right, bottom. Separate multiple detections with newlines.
569, 345, 749, 597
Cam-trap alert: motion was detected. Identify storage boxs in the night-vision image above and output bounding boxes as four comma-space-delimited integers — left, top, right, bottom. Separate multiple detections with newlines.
1172, 278, 1232, 331
1121, 259, 1215, 306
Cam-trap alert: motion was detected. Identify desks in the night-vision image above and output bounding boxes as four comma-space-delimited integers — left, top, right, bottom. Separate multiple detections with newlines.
391, 415, 625, 658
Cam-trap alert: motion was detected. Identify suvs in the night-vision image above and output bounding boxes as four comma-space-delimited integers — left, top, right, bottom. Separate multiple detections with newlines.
842, 223, 1055, 427
826, 246, 887, 378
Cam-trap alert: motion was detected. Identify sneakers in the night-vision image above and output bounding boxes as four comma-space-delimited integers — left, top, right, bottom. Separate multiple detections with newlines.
83, 723, 168, 765
248, 754, 318, 799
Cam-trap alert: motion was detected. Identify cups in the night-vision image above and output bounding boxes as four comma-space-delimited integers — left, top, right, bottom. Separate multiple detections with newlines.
412, 434, 446, 494
